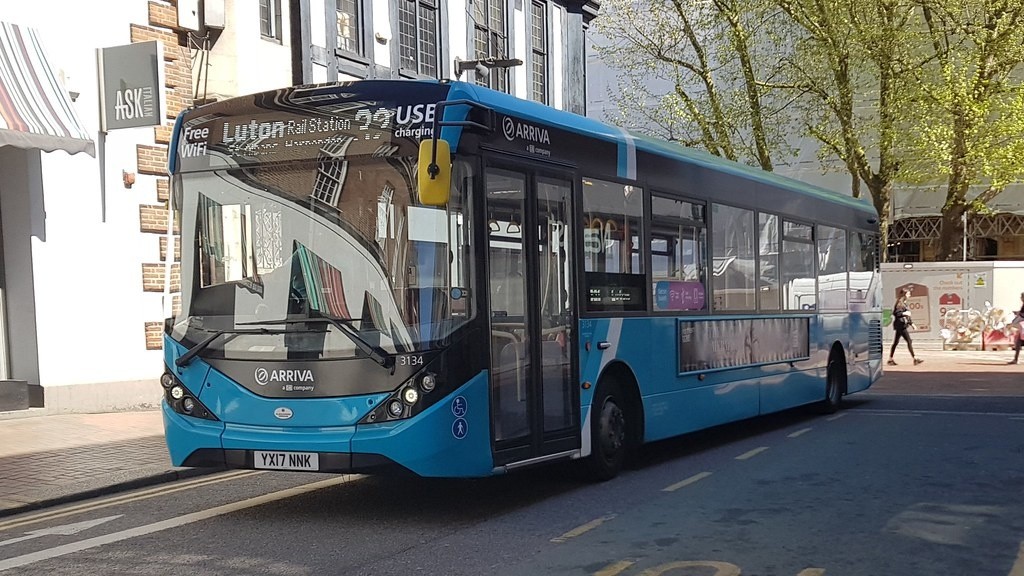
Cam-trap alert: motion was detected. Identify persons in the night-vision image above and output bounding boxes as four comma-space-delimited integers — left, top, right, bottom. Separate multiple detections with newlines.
888, 289, 924, 367
1008, 292, 1024, 364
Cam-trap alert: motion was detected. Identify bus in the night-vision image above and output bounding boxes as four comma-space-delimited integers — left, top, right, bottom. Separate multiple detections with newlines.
161, 80, 887, 478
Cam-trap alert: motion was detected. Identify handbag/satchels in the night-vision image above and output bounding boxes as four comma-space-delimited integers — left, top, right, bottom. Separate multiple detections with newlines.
896, 317, 913, 326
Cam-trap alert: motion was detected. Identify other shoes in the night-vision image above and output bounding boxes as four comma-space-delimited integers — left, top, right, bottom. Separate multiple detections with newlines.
1008, 360, 1017, 364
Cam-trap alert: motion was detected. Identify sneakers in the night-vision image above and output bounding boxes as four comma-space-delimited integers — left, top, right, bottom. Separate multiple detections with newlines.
888, 359, 898, 365
914, 359, 924, 365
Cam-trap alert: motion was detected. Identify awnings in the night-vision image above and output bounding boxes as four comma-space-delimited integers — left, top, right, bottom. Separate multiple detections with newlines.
0, 21, 96, 159
